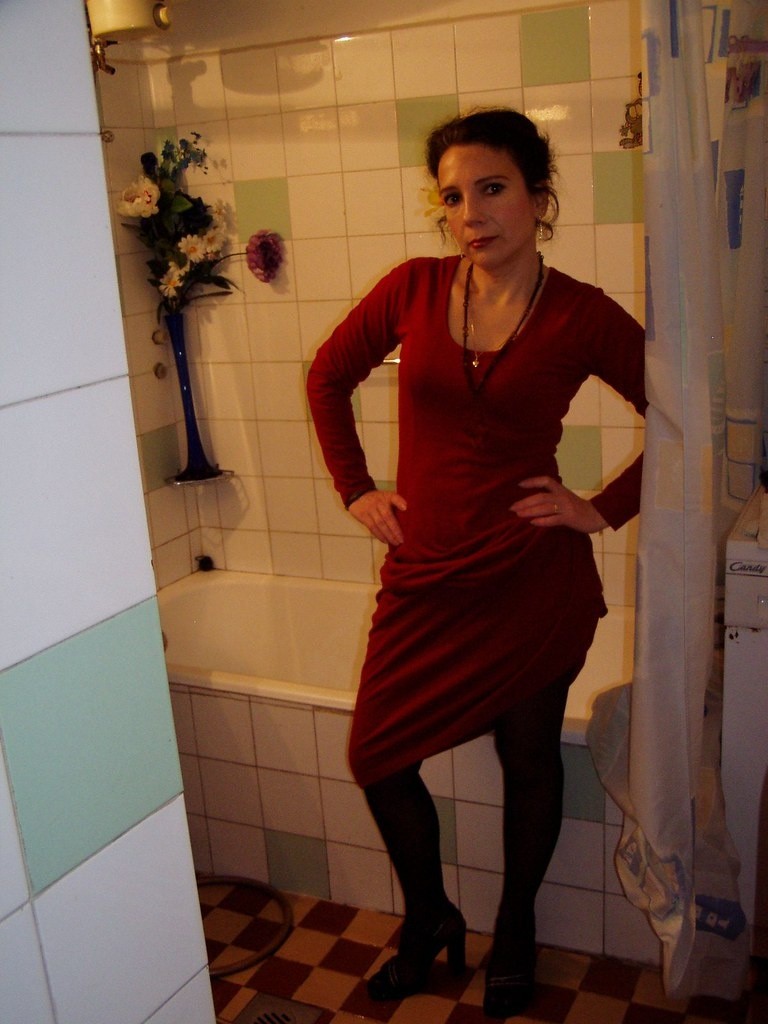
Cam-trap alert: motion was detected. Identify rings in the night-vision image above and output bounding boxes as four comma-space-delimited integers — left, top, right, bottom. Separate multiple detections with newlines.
554, 504, 559, 513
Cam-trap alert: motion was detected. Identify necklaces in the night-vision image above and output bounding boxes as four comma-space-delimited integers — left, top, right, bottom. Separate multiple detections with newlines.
463, 250, 544, 397
468, 301, 509, 367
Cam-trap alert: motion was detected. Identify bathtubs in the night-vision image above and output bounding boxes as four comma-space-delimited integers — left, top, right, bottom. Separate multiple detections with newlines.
157, 567, 634, 749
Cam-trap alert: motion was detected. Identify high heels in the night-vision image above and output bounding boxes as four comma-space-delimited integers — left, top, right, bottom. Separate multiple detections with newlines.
482, 908, 536, 1019
367, 897, 469, 1001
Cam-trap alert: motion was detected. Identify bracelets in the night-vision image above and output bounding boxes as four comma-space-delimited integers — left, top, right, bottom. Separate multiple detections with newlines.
344, 485, 378, 511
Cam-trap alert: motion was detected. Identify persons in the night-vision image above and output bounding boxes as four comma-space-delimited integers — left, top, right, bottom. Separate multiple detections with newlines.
306, 104, 650, 1019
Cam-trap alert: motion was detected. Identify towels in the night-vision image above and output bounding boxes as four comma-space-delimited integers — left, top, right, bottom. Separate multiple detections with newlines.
584, 681, 750, 1001
714, 0, 768, 512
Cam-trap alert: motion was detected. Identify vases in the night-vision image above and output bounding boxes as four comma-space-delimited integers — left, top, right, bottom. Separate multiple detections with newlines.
164, 311, 224, 482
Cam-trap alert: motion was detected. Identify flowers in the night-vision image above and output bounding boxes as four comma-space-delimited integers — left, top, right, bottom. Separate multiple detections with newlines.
113, 132, 284, 323
